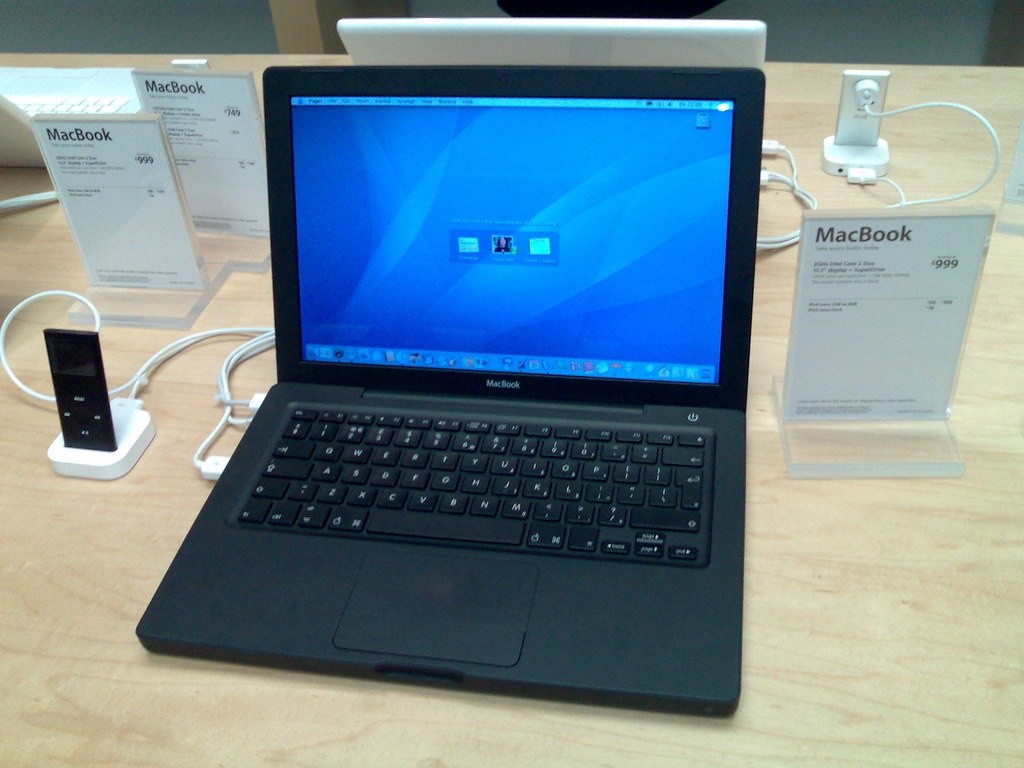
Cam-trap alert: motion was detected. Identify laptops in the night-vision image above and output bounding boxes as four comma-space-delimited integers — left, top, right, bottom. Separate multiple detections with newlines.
336, 17, 768, 70
0, 67, 144, 169
135, 66, 765, 718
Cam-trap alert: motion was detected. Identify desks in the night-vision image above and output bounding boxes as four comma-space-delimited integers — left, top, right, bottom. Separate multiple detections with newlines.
0, 51, 1024, 768
